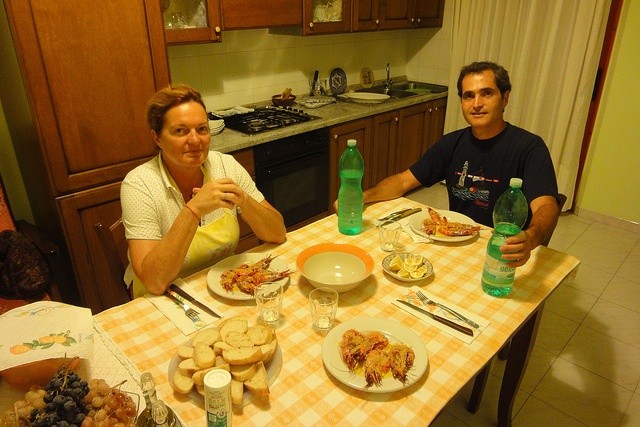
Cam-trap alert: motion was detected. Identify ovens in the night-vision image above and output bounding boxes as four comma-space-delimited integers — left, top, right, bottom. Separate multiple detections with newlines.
255, 127, 329, 227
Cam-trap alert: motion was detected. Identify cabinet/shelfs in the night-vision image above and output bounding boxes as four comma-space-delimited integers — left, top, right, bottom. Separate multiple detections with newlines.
303, 1, 381, 37
380, 0, 447, 30
398, 99, 450, 211
3, 1, 171, 310
160, 1, 223, 46
325, 113, 396, 214
228, 148, 261, 253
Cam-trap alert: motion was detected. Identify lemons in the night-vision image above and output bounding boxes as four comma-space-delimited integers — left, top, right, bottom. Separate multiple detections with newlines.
389, 253, 428, 279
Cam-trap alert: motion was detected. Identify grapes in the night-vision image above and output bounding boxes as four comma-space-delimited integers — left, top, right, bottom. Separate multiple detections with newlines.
18, 352, 91, 427
1, 389, 48, 427
81, 378, 137, 427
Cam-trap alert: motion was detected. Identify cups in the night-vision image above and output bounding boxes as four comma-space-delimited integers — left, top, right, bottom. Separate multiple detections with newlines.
309, 288, 339, 333
172, 5, 185, 28
254, 281, 282, 324
379, 220, 402, 253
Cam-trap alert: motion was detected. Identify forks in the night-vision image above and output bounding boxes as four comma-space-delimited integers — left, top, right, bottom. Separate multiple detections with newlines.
415, 291, 479, 328
376, 210, 410, 222
163, 291, 199, 321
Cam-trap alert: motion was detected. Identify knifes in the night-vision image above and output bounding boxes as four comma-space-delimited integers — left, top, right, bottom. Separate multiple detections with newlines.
397, 299, 474, 336
168, 284, 222, 318
377, 207, 422, 229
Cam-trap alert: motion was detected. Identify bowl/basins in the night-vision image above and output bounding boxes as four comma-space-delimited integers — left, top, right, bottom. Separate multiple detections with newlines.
272, 95, 297, 105
296, 244, 374, 294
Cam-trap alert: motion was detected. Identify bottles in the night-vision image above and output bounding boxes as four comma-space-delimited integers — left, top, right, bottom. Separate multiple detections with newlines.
337, 138, 364, 235
481, 178, 529, 298
141, 372, 184, 426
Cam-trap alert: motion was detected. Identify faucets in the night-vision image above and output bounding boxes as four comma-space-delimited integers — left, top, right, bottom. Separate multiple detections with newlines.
385, 62, 393, 89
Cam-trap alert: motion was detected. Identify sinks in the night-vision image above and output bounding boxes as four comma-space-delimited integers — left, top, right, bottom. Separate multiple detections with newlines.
364, 87, 416, 100
396, 82, 442, 93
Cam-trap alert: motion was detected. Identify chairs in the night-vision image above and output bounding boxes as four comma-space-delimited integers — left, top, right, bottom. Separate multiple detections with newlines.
94, 219, 133, 301
468, 194, 569, 413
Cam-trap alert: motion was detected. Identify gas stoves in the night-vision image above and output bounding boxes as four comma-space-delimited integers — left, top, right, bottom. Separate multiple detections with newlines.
207, 105, 322, 136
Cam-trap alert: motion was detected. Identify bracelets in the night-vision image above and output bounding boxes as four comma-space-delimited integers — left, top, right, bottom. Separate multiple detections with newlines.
184, 206, 201, 227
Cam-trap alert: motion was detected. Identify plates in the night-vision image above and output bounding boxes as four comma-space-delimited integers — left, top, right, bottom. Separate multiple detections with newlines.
168, 326, 281, 413
347, 92, 390, 105
0, 390, 140, 427
209, 120, 225, 137
207, 253, 289, 301
382, 252, 433, 282
321, 315, 427, 394
360, 66, 374, 88
408, 210, 479, 243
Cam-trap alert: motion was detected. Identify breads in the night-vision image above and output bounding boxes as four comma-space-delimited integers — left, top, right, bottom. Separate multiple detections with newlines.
172, 315, 278, 406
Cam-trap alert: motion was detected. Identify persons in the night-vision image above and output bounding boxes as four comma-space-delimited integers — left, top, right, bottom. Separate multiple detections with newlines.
332, 61, 559, 267
119, 86, 287, 298
